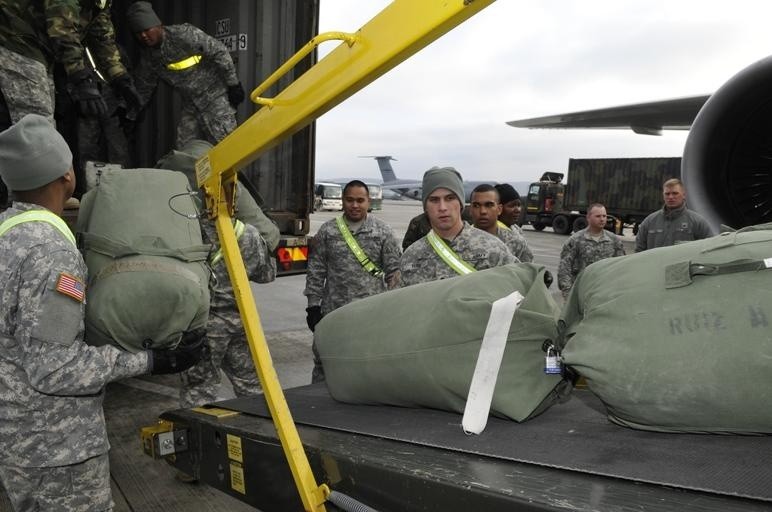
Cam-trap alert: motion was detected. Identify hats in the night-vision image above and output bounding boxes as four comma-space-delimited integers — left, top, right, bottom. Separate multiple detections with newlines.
423, 167, 465, 213
0, 114, 73, 192
126, 0, 162, 31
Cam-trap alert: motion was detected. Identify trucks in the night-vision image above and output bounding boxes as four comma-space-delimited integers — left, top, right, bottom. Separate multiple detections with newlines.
525, 157, 680, 236
314, 183, 383, 213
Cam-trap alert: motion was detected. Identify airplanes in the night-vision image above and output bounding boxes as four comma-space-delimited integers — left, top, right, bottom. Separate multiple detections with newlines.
506, 93, 713, 136
357, 155, 500, 203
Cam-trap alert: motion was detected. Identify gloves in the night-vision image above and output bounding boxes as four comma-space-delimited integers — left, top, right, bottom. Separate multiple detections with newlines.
228, 83, 245, 106
69, 69, 108, 115
110, 74, 142, 107
151, 328, 207, 373
111, 106, 127, 120
305, 306, 323, 331
119, 118, 136, 135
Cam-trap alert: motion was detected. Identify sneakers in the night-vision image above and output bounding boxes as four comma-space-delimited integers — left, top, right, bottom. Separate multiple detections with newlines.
64, 198, 79, 210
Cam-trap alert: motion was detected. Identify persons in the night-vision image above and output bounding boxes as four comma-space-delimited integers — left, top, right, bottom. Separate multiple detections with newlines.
388, 166, 521, 293
494, 183, 525, 235
125, 1, 245, 145
75, 38, 132, 168
1, 113, 209, 511
1, 1, 145, 209
557, 203, 628, 302
634, 179, 714, 252
303, 180, 402, 384
402, 207, 475, 252
178, 184, 277, 483
470, 183, 536, 264
